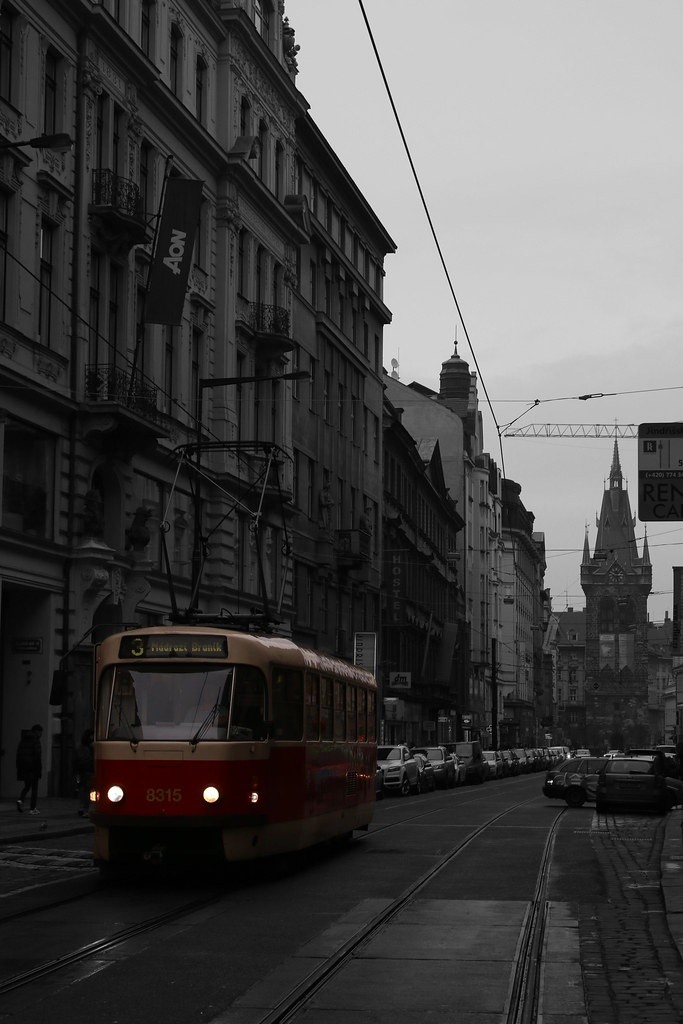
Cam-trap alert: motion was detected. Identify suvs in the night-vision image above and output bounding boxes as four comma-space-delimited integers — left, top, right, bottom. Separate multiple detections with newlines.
543, 756, 663, 814
377, 745, 466, 794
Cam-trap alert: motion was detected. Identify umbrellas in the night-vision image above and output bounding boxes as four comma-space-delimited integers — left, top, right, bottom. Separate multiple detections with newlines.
64, 734, 82, 769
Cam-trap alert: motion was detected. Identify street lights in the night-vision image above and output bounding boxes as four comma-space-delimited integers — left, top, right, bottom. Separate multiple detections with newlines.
189, 371, 311, 613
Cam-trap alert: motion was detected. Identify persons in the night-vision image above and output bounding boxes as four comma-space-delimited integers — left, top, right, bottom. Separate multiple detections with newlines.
186, 683, 228, 729
16, 724, 44, 816
74, 727, 94, 818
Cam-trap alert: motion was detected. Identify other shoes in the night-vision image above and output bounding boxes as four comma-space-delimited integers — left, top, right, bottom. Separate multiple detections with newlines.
78, 808, 90, 818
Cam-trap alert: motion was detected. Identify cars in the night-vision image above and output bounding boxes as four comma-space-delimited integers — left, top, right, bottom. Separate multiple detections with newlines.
482, 746, 571, 777
575, 749, 591, 757
603, 750, 625, 757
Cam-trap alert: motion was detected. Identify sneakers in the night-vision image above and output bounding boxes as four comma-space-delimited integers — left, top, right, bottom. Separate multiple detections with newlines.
17, 800, 24, 813
28, 808, 40, 815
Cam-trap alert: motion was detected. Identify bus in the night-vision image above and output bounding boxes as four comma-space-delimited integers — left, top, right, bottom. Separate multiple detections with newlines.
92, 627, 379, 860
